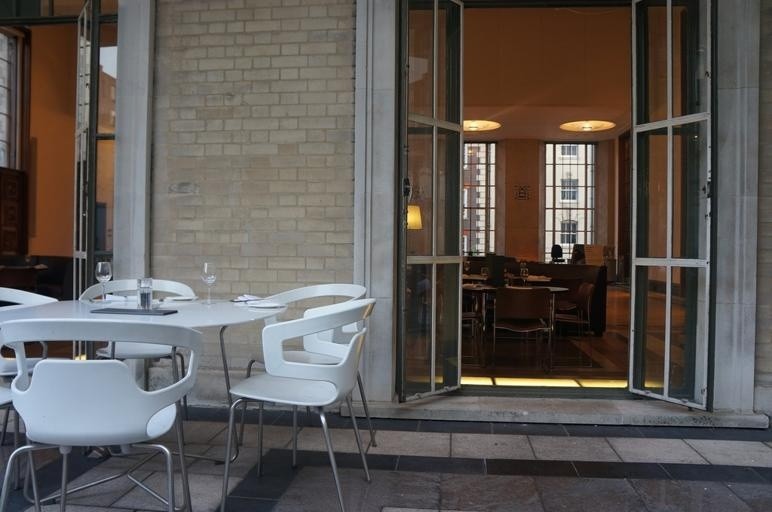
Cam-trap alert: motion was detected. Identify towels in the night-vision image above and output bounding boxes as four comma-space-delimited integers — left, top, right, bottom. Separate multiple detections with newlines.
235, 294, 282, 308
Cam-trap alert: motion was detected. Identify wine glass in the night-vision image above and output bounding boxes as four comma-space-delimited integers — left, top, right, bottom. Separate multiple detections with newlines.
519, 262, 526, 268
95, 261, 112, 302
200, 262, 218, 306
481, 267, 488, 279
463, 261, 471, 271
521, 268, 528, 287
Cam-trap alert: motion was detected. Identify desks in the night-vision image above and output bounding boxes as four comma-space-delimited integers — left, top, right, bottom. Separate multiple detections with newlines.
462, 273, 490, 284
506, 274, 552, 282
463, 284, 569, 331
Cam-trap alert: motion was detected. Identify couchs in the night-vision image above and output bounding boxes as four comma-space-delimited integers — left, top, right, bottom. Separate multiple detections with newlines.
462, 255, 515, 273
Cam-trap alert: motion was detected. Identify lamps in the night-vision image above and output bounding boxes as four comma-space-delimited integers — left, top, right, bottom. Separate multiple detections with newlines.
463, 120, 502, 132
560, 120, 616, 132
406, 205, 423, 231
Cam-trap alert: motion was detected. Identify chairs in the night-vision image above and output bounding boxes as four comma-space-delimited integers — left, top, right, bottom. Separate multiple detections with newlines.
78, 279, 196, 423
462, 312, 485, 366
551, 283, 595, 335
0, 287, 60, 445
491, 287, 552, 359
221, 298, 377, 512
0, 316, 203, 510
552, 245, 565, 263
237, 284, 378, 468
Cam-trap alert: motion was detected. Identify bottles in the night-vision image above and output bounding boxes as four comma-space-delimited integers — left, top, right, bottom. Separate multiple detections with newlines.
136, 277, 153, 311
